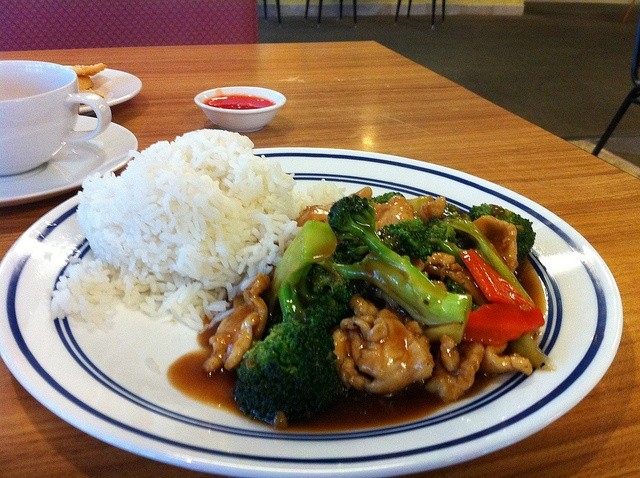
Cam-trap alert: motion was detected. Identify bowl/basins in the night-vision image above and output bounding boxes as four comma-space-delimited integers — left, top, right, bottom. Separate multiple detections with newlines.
193, 84, 289, 132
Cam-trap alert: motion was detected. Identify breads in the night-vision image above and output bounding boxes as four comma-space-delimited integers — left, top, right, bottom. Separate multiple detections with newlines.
72, 64, 109, 93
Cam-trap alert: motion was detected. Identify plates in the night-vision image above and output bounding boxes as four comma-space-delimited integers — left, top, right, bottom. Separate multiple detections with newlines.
67, 63, 144, 115
0, 149, 627, 476
1, 111, 139, 207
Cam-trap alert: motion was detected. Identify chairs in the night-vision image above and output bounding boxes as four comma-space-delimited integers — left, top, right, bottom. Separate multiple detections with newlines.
591, 27, 640, 157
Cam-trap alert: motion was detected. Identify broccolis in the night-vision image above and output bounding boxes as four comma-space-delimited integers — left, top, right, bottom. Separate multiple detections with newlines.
234, 282, 344, 429
316, 191, 552, 372
266, 221, 336, 300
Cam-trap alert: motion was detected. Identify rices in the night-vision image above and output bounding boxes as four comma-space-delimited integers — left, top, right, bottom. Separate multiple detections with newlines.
43, 128, 347, 334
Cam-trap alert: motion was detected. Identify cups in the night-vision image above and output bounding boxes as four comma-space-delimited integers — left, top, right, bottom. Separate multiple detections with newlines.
0, 58, 109, 177
